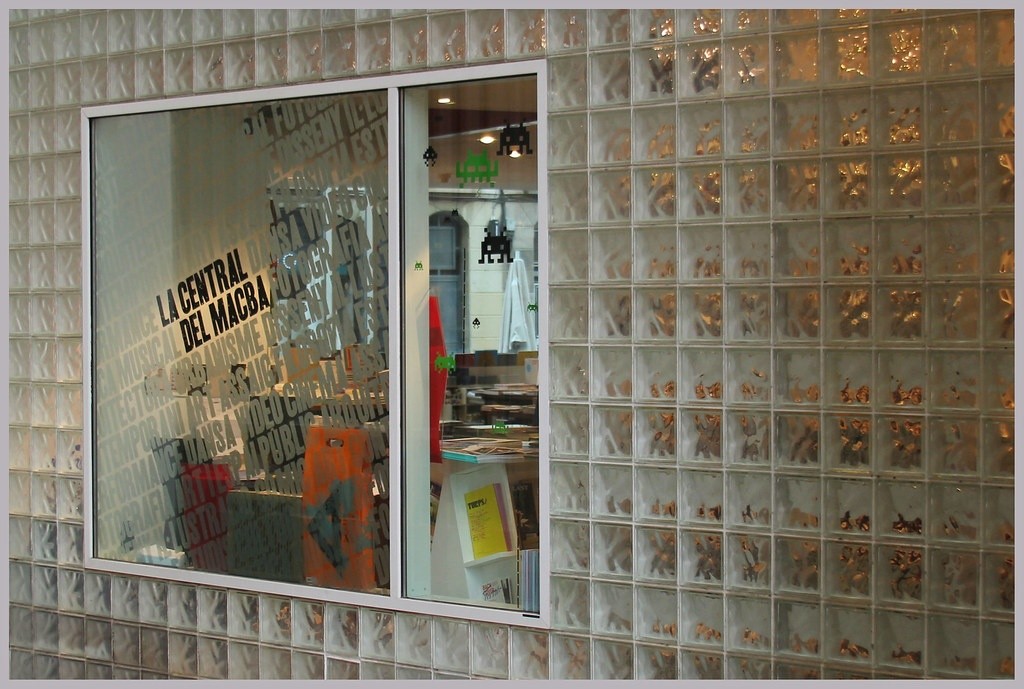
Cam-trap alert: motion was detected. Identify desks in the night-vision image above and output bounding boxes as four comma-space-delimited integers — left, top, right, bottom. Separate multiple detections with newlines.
247, 383, 539, 548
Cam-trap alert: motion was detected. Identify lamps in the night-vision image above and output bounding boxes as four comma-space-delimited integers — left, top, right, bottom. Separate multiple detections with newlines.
489, 220, 500, 237
509, 125, 521, 158
